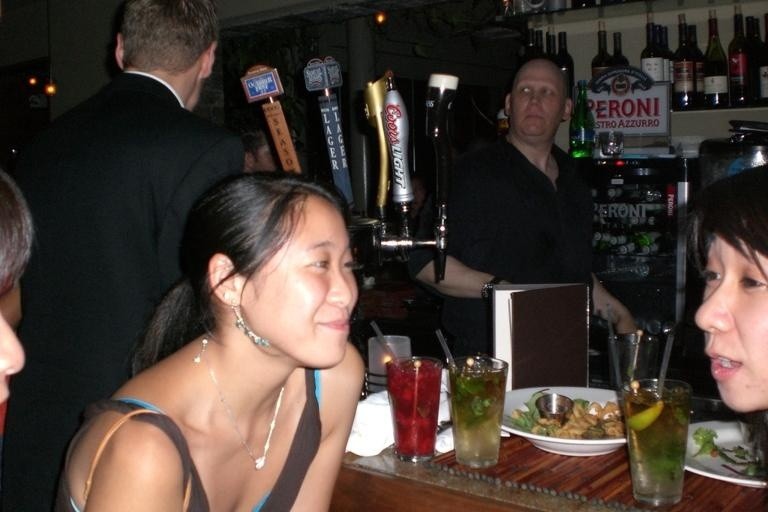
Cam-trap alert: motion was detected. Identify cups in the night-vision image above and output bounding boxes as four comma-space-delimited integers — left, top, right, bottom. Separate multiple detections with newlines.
534, 395, 573, 426
620, 380, 691, 506
384, 354, 443, 462
446, 354, 509, 468
369, 335, 411, 396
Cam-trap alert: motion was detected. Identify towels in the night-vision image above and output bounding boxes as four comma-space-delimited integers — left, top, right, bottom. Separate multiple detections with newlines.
346, 368, 451, 457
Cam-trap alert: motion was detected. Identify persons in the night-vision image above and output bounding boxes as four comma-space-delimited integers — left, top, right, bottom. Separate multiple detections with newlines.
0, 2, 365, 512
407, 56, 635, 367
683, 164, 768, 412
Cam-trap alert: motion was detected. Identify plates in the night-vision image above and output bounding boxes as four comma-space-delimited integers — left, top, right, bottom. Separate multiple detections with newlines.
685, 420, 767, 490
504, 387, 628, 456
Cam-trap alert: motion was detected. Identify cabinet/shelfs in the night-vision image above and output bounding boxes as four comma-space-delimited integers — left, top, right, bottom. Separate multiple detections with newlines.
527, 0, 768, 158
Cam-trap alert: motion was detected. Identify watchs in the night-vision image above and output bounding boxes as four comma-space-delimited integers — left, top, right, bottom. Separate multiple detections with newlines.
481, 275, 504, 301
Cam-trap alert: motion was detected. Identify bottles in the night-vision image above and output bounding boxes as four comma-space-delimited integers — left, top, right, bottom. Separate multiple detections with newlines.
608, 331, 675, 387
571, 81, 596, 161
585, 175, 671, 258
501, 0, 768, 105
